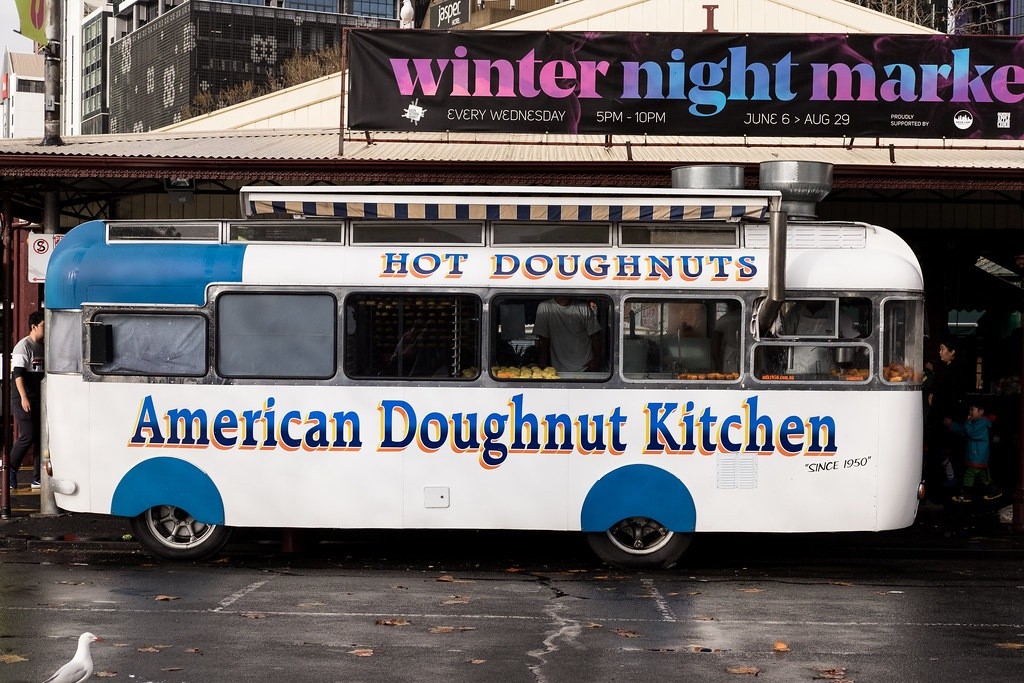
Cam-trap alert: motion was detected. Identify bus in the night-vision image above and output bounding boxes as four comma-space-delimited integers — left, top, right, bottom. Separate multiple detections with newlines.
28, 185, 926, 572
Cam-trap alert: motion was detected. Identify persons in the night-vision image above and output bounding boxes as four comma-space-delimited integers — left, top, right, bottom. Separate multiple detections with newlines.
711, 299, 743, 374
532, 295, 603, 372
9, 311, 46, 490
923, 337, 1004, 504
774, 299, 867, 374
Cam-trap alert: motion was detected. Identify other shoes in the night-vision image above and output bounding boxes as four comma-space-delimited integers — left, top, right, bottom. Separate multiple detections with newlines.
31, 480, 41, 489
943, 479, 957, 486
983, 490, 1002, 500
10, 469, 18, 489
952, 492, 972, 503
